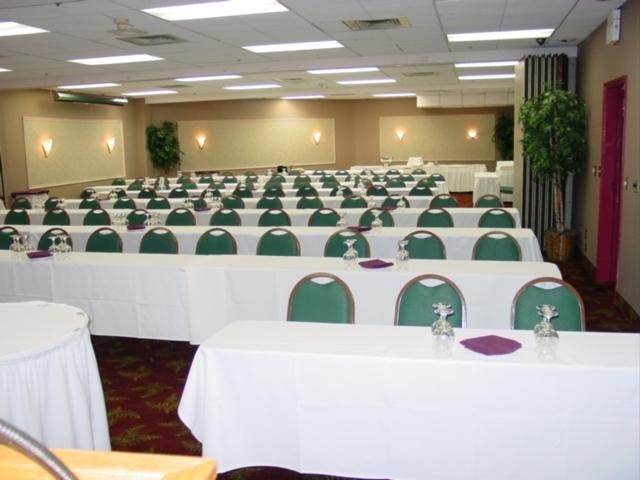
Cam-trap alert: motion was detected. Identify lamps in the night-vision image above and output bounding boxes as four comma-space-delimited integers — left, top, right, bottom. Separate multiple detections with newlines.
195, 135, 206, 150
106, 137, 115, 153
467, 130, 476, 138
42, 139, 52, 158
395, 129, 404, 141
311, 132, 321, 145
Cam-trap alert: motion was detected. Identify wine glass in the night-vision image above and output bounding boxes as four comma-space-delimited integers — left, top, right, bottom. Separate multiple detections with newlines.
357, 179, 364, 189
431, 303, 454, 340
367, 194, 376, 208
534, 305, 559, 345
343, 239, 358, 264
205, 189, 213, 201
396, 239, 408, 263
335, 186, 343, 196
111, 213, 129, 226
55, 198, 65, 209
349, 174, 355, 182
109, 188, 118, 200
396, 194, 406, 209
181, 198, 194, 210
144, 213, 162, 225
8, 234, 26, 257
335, 211, 347, 230
370, 210, 383, 231
48, 235, 71, 259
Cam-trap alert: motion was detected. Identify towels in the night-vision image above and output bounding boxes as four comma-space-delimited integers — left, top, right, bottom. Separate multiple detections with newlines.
458, 334, 522, 355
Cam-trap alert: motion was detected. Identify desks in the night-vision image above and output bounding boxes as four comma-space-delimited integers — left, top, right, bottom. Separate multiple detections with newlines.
177, 320, 640, 480
0, 301, 113, 451
0, 443, 217, 480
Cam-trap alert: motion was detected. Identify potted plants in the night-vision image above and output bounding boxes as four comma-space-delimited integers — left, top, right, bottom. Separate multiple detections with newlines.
518, 88, 590, 263
